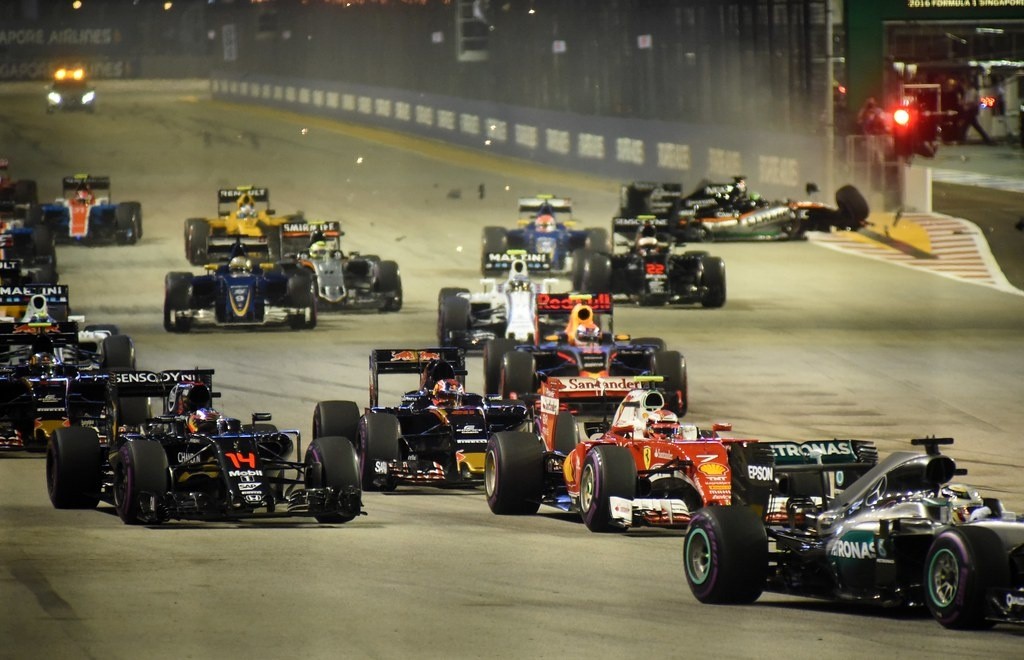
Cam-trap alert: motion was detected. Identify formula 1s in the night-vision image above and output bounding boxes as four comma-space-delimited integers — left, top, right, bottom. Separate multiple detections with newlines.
484, 375, 758, 532
45, 367, 368, 524
165, 186, 403, 330
437, 249, 574, 354
683, 434, 1024, 630
485, 292, 688, 417
0, 156, 150, 453
312, 347, 535, 493
480, 176, 870, 308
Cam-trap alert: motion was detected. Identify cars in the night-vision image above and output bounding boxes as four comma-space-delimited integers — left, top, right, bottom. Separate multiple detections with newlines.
42, 67, 97, 115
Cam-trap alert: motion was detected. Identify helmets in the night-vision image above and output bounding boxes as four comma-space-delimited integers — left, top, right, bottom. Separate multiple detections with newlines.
75, 191, 92, 205
309, 240, 329, 258
535, 215, 557, 233
31, 314, 52, 322
646, 410, 680, 440
30, 353, 57, 379
238, 204, 258, 219
638, 236, 659, 254
189, 408, 222, 434
941, 483, 985, 526
432, 380, 464, 406
228, 257, 252, 273
575, 323, 603, 347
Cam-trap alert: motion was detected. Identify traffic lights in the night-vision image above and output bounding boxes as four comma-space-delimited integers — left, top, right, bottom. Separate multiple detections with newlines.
892, 104, 921, 156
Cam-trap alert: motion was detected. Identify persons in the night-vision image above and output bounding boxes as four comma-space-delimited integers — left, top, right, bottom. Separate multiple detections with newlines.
857, 98, 884, 134
646, 410, 700, 439
432, 379, 465, 407
30, 352, 63, 377
940, 484, 991, 524
310, 241, 342, 261
536, 215, 556, 232
76, 189, 96, 205
240, 205, 257, 218
575, 323, 602, 347
188, 408, 241, 435
229, 256, 252, 275
640, 238, 657, 257
